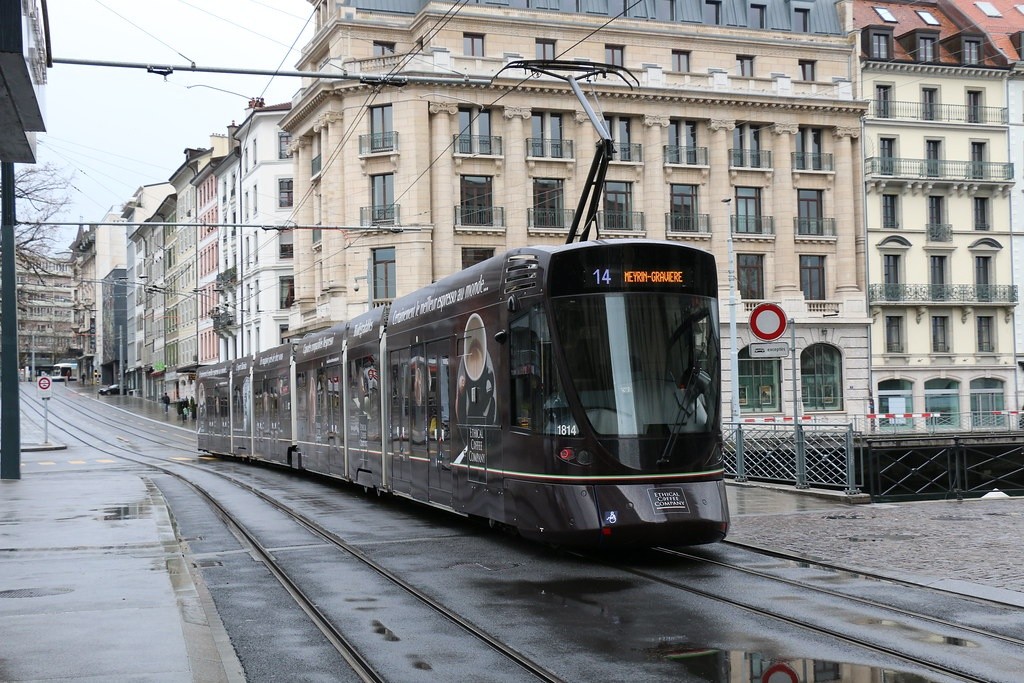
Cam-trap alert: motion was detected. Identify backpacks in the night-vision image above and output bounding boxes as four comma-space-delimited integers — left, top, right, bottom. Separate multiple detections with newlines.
164, 397, 169, 403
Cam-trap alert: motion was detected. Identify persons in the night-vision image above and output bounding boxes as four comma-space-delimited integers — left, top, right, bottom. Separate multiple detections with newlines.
178, 400, 185, 422
81, 373, 86, 384
66, 370, 70, 381
163, 392, 170, 413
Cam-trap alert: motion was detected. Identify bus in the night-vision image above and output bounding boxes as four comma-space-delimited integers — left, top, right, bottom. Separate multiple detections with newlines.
51, 363, 78, 381
195, 239, 730, 552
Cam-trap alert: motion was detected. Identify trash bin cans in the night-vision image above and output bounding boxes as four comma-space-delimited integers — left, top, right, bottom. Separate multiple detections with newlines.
175, 399, 188, 420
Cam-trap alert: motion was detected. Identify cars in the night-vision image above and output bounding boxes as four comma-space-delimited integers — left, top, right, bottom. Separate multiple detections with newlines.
99, 385, 131, 396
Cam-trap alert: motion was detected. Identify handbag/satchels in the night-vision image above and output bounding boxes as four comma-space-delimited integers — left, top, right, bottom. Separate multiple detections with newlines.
184, 415, 186, 420
177, 414, 182, 420
